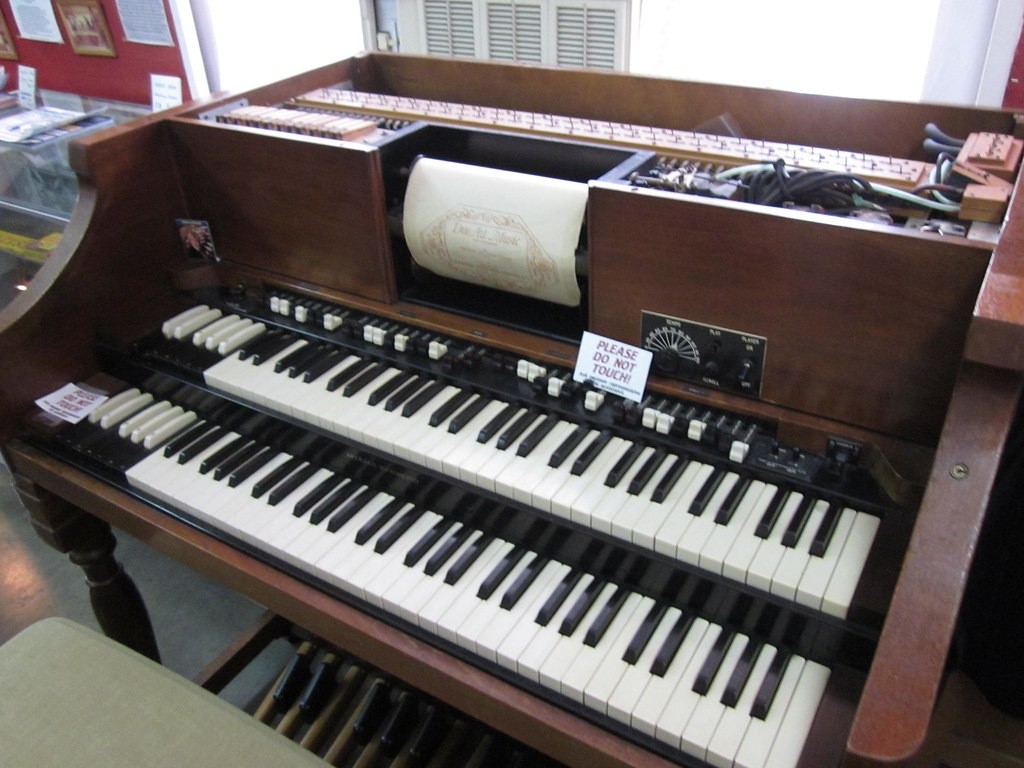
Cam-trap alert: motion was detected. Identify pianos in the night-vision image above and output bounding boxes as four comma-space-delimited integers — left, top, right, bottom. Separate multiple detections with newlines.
0, 52, 1022, 768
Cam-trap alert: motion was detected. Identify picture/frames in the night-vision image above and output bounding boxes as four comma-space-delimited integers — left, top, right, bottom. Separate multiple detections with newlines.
0, 9, 18, 60
55, 0, 117, 58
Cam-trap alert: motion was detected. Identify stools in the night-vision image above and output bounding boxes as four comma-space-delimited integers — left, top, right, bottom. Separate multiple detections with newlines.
0, 618, 338, 768
